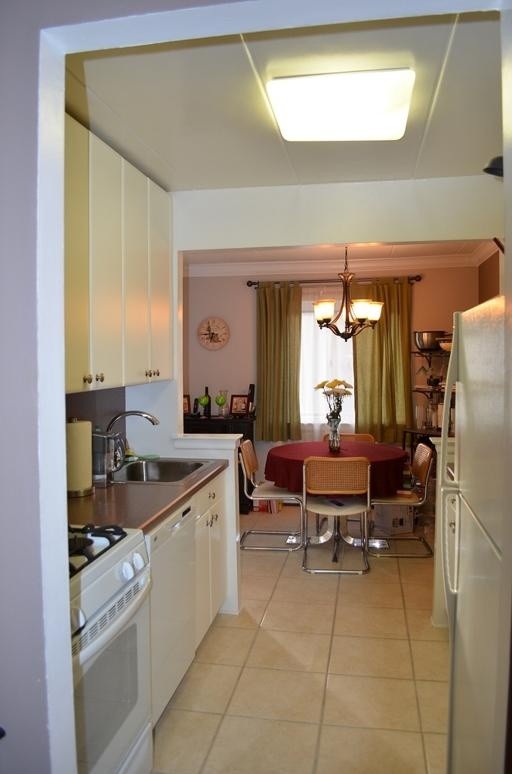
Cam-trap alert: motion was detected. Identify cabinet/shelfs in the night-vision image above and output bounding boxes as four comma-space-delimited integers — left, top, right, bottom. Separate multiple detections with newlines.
401, 349, 456, 479
123, 157, 176, 393
135, 498, 197, 729
195, 469, 229, 650
64, 111, 123, 395
184, 414, 257, 514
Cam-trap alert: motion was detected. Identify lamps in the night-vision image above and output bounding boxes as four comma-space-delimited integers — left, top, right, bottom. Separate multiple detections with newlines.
312, 246, 384, 343
265, 66, 418, 144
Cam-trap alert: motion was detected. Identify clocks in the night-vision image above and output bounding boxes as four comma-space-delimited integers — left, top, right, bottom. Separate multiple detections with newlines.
197, 316, 231, 352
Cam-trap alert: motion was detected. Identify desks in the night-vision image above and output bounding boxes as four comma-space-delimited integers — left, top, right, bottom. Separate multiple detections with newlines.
380, 465, 380, 465
264, 441, 411, 549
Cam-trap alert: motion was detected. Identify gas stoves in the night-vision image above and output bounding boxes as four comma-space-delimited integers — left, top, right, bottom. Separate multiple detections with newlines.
68, 520, 127, 578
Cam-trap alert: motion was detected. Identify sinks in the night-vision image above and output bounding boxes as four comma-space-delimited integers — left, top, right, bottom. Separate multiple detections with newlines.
110, 457, 215, 486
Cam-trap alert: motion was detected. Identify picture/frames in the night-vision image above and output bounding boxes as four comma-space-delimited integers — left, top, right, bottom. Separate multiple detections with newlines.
183, 394, 191, 416
229, 394, 249, 417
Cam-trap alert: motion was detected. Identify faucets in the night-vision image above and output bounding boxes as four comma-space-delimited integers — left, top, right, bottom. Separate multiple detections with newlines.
106, 410, 160, 434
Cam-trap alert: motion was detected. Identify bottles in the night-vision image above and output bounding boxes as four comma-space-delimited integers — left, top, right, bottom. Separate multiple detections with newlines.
203, 386, 212, 418
430, 403, 438, 428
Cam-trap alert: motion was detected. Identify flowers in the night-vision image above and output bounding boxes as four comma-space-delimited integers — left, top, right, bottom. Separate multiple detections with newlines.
314, 378, 354, 448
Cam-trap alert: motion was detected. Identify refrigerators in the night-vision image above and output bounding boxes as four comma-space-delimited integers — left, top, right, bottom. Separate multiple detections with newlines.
439, 295, 512, 774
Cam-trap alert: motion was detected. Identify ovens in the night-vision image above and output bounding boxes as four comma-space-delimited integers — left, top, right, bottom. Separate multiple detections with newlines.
72, 543, 155, 774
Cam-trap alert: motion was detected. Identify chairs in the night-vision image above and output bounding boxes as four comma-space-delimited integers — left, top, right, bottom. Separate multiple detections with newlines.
370, 442, 435, 559
302, 455, 372, 575
316, 432, 376, 534
237, 439, 306, 553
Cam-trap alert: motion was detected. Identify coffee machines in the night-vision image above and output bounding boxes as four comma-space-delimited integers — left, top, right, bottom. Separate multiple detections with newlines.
93, 431, 117, 488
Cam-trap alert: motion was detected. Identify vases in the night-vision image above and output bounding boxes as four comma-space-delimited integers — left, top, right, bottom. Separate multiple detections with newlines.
327, 413, 342, 451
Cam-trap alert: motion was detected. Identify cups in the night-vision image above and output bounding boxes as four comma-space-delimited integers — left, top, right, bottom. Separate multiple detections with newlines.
218, 390, 229, 417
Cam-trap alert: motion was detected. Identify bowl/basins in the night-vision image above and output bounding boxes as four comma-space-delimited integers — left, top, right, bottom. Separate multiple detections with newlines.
435, 337, 452, 351
414, 330, 446, 350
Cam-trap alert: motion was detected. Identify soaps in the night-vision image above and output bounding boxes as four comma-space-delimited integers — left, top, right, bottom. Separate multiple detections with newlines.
142, 454, 160, 460
